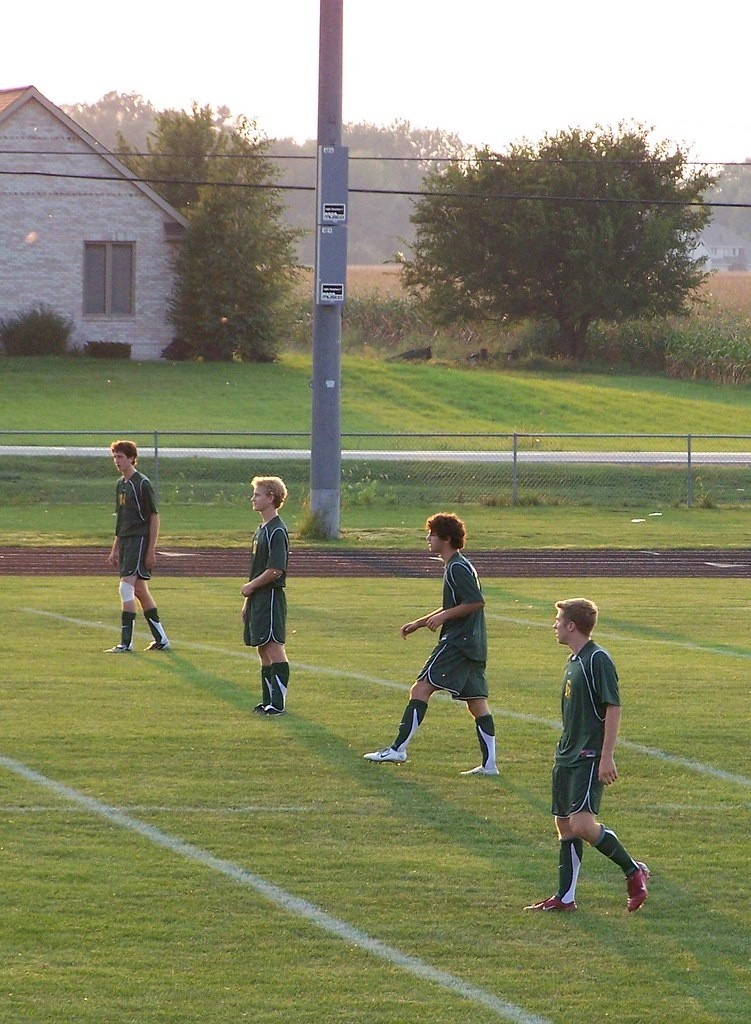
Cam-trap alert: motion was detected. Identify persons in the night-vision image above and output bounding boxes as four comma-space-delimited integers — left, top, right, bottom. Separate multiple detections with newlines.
523, 597, 653, 916
239, 475, 291, 717
362, 512, 502, 777
102, 440, 170, 652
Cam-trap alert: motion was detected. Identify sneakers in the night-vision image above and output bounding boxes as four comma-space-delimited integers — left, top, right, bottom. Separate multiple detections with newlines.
523, 895, 577, 912
625, 861, 651, 913
252, 703, 285, 717
364, 747, 407, 766
460, 765, 499, 776
103, 644, 134, 653
143, 642, 170, 652
